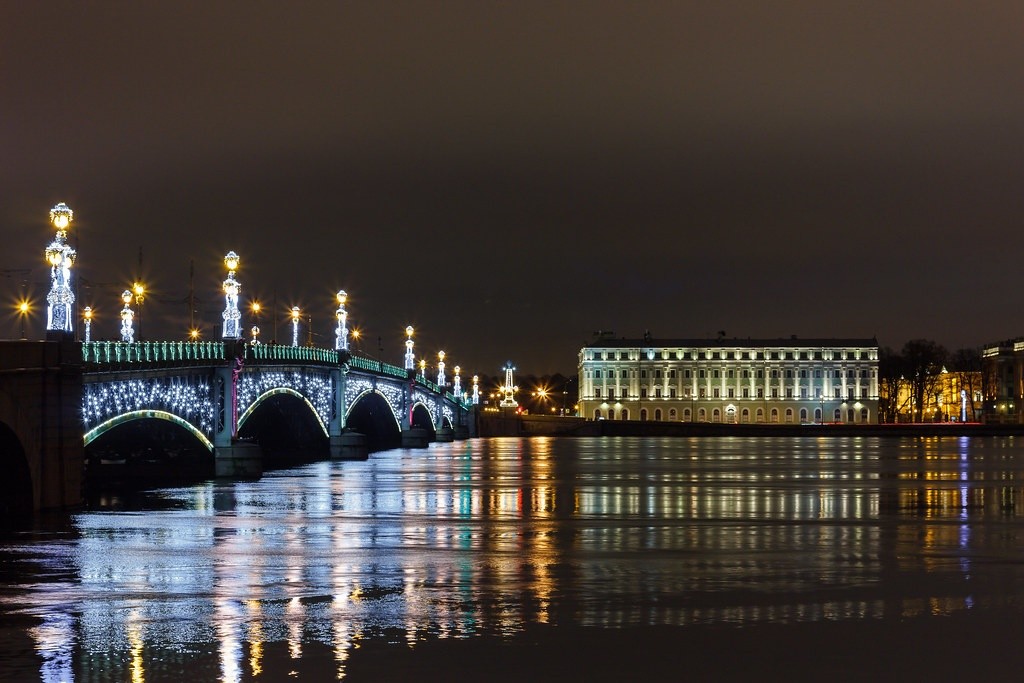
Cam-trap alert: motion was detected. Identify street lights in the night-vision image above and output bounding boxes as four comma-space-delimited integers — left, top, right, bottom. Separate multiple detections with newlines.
353, 331, 359, 357
455, 366, 460, 396
405, 326, 414, 368
120, 290, 136, 344
292, 306, 300, 346
253, 303, 259, 343
223, 250, 242, 337
439, 351, 446, 386
335, 290, 349, 349
46, 203, 76, 331
134, 283, 144, 339
820, 395, 823, 424
19, 302, 29, 338
472, 375, 478, 404
635, 396, 641, 420
420, 360, 426, 378
84, 307, 92, 342
